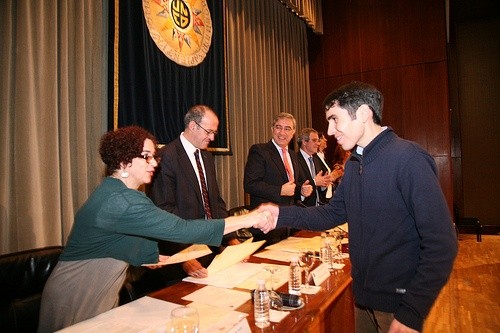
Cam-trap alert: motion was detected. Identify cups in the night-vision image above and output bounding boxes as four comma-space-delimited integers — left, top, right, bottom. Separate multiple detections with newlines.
170, 307, 199, 333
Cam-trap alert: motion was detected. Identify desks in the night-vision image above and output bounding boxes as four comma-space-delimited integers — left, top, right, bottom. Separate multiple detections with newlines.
52, 222, 358, 333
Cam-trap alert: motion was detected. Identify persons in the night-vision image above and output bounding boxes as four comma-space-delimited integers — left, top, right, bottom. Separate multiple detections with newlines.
476, 219, 482, 242
39, 127, 274, 333
145, 105, 351, 286
252, 81, 459, 333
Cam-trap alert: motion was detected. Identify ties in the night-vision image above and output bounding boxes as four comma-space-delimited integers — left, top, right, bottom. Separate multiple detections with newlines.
281, 147, 293, 182
309, 157, 320, 206
194, 149, 212, 220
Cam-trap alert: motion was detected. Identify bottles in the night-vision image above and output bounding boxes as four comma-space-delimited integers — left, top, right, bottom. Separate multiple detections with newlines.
253, 281, 270, 327
288, 257, 302, 295
320, 232, 333, 269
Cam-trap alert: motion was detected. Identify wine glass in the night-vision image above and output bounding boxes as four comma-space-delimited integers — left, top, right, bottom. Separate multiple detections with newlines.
328, 229, 346, 263
299, 249, 315, 291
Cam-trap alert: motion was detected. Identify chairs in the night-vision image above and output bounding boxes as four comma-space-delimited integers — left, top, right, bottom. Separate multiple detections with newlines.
454, 204, 483, 242
0, 204, 255, 333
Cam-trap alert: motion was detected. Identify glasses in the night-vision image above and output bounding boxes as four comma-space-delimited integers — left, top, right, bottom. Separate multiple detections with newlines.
139, 154, 162, 164
193, 120, 218, 135
